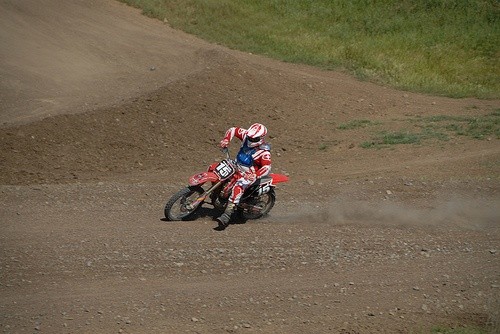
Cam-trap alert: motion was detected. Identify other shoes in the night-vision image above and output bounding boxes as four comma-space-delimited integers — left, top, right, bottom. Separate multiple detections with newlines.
216, 208, 233, 229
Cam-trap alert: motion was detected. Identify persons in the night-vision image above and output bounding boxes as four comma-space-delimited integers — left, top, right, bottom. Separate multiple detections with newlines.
216, 123, 272, 229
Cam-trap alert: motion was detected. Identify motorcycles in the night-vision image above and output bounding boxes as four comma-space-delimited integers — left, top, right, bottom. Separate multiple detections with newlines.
163, 140, 290, 221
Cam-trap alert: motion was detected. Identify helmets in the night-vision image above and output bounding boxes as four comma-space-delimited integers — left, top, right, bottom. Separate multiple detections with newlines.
245, 123, 267, 147
